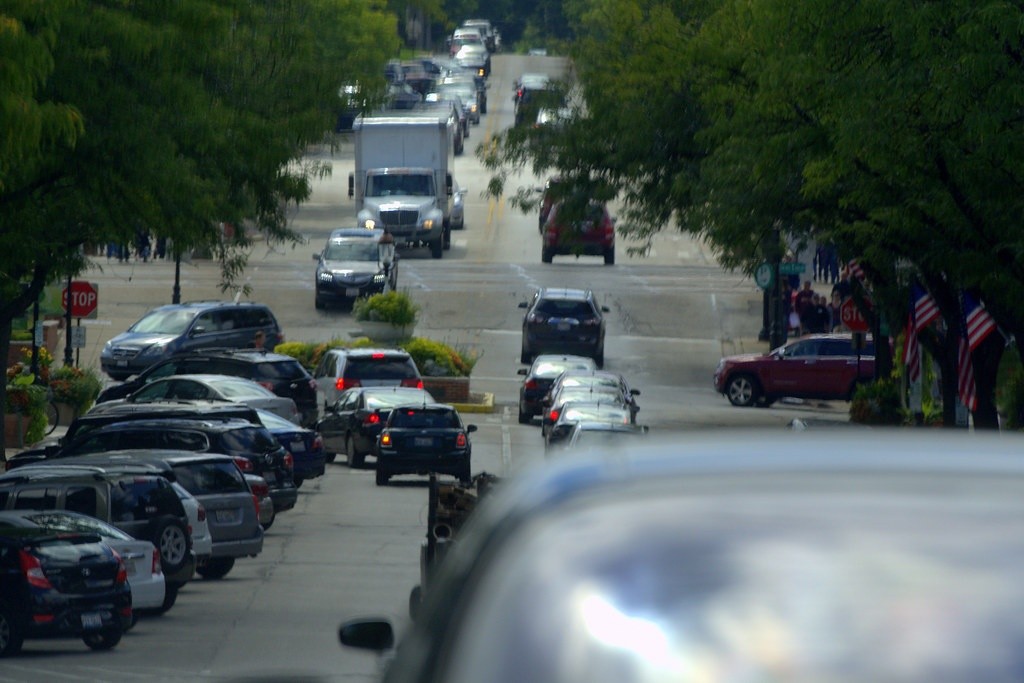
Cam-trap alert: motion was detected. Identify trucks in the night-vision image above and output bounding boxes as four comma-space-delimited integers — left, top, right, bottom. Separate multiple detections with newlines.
352, 113, 459, 257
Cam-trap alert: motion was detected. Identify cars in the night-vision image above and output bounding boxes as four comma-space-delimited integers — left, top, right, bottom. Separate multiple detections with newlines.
310, 385, 438, 464
447, 175, 468, 230
369, 402, 478, 493
511, 354, 656, 448
253, 409, 328, 487
335, 421, 1023, 679
339, 13, 578, 142
310, 228, 401, 312
0, 526, 136, 656
0, 509, 166, 626
86, 372, 303, 436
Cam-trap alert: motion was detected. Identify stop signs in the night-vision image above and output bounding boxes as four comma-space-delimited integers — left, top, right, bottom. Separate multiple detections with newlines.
842, 297, 885, 334
61, 281, 101, 320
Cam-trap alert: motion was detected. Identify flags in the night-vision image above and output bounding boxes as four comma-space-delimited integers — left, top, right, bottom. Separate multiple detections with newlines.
957, 282, 997, 414
900, 275, 942, 382
847, 249, 864, 276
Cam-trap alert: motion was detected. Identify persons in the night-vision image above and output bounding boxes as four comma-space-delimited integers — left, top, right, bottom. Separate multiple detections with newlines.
784, 240, 872, 336
253, 331, 270, 352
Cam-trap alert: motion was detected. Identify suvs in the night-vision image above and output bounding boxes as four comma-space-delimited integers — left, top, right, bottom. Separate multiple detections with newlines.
536, 175, 604, 237
6, 419, 293, 530
710, 332, 899, 409
0, 464, 197, 613
310, 345, 425, 401
25, 399, 264, 436
99, 301, 285, 381
514, 285, 614, 370
539, 197, 617, 264
9, 449, 268, 579
94, 346, 321, 436
